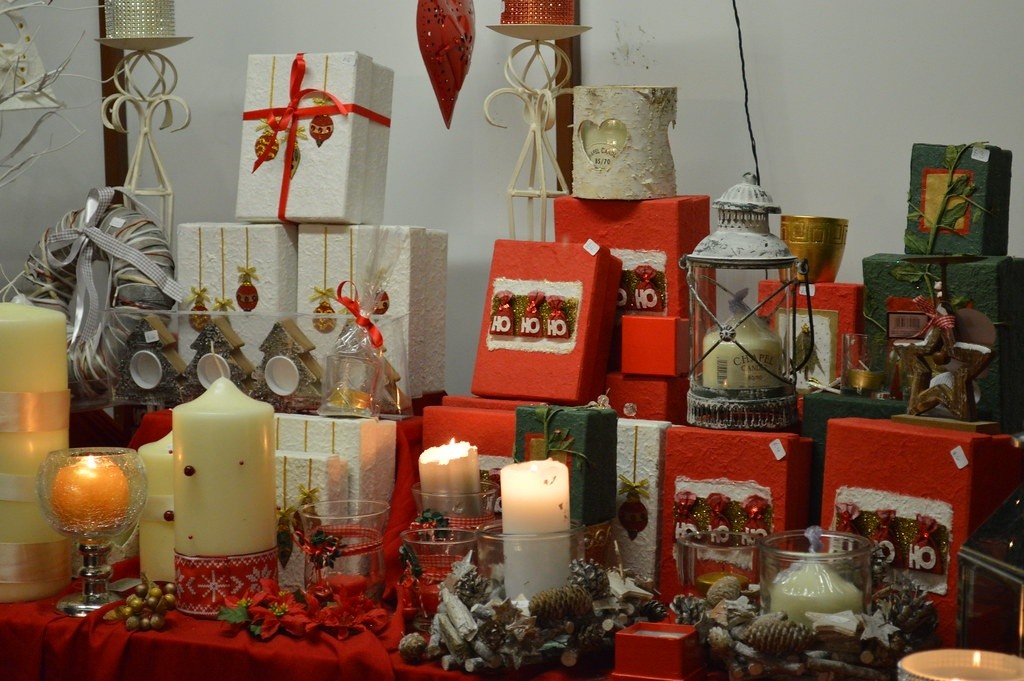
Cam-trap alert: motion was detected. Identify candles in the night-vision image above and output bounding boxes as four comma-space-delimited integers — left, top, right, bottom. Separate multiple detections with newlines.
171, 338, 284, 619
50, 452, 130, 523
497, 455, 570, 604
419, 440, 486, 521
772, 525, 865, 624
134, 428, 177, 583
1, 300, 70, 602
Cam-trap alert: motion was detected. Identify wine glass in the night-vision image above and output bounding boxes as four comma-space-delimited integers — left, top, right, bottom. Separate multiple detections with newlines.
36, 446, 152, 617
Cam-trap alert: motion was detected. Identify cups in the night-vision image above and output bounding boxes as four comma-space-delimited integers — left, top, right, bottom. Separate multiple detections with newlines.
840, 333, 896, 399
758, 530, 872, 628
298, 498, 386, 612
474, 517, 586, 610
896, 648, 1024, 681
674, 529, 762, 610
411, 480, 498, 573
321, 356, 380, 420
104, 1, 176, 37
778, 214, 850, 284
500, 1, 576, 26
571, 85, 677, 201
401, 528, 477, 631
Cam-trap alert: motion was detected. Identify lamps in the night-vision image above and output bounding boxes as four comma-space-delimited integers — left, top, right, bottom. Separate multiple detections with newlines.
673, 172, 802, 432
